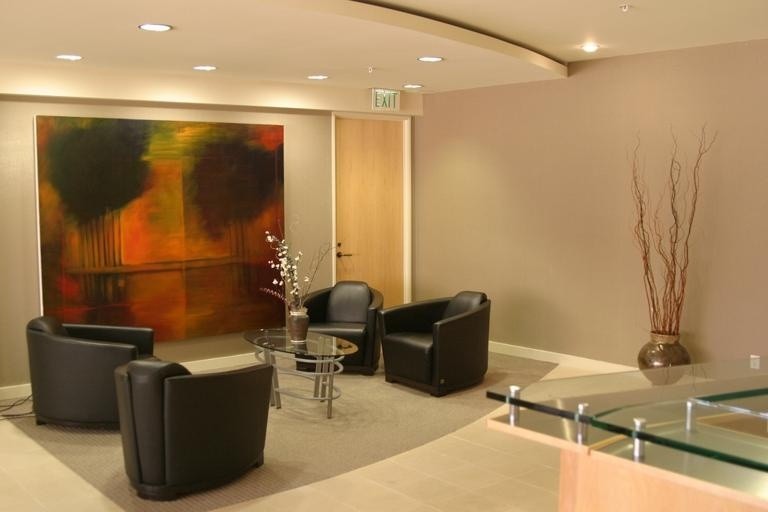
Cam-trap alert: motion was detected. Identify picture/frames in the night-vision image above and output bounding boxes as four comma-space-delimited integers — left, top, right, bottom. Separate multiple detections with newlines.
34, 116, 286, 343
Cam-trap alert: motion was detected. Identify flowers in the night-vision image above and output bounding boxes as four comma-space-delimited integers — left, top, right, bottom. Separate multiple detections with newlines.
259, 217, 337, 311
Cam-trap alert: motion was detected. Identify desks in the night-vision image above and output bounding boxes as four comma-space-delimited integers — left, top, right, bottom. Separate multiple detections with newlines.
487, 361, 768, 512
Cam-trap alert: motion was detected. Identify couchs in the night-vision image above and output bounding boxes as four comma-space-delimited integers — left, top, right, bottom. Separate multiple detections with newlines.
295, 281, 384, 376
378, 291, 491, 398
113, 357, 274, 501
26, 315, 154, 430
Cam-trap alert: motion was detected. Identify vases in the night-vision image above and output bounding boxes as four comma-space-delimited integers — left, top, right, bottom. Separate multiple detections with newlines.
288, 312, 310, 343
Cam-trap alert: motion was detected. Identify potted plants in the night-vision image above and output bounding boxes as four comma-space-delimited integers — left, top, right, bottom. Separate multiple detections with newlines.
624, 122, 721, 385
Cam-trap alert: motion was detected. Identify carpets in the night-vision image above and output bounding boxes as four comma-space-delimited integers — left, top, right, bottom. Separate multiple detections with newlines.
0, 351, 558, 512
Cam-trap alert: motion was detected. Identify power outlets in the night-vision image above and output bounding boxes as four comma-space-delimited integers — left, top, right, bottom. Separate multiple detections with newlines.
750, 355, 760, 369
